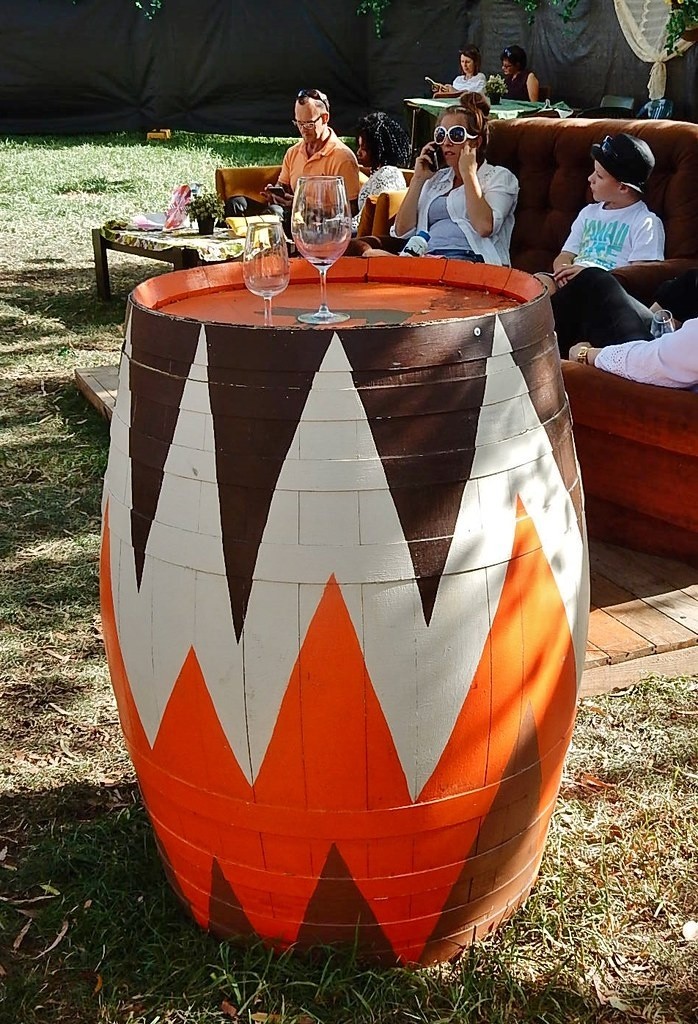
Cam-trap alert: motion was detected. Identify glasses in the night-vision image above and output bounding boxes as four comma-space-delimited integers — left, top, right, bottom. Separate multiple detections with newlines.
292, 115, 323, 129
297, 90, 330, 112
504, 48, 516, 60
432, 126, 481, 145
602, 134, 618, 159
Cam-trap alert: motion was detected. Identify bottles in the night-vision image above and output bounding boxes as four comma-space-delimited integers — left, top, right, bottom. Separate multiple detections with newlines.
682, 920, 697, 940
399, 231, 431, 257
191, 183, 198, 200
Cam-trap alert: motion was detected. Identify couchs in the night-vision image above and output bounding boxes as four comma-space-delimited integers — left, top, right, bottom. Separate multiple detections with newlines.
390, 118, 698, 565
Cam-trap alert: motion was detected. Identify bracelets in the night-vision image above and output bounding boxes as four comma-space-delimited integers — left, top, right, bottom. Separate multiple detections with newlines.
577, 346, 593, 365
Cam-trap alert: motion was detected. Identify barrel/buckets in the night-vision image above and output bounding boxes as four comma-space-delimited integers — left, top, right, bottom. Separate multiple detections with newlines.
100, 259, 591, 966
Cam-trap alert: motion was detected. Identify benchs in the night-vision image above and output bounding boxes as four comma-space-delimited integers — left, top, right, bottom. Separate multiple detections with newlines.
216, 166, 415, 203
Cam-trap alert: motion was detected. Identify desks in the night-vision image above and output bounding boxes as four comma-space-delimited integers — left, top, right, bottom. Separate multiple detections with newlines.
91, 227, 295, 303
404, 95, 583, 154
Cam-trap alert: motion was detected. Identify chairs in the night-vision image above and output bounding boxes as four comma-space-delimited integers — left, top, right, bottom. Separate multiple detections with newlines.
576, 94, 635, 118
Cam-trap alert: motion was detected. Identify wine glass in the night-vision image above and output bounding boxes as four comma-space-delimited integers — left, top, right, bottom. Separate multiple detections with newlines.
290, 175, 352, 325
243, 222, 290, 327
650, 309, 676, 338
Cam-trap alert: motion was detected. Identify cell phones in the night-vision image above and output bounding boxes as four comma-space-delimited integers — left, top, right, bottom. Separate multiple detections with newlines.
269, 187, 288, 201
433, 148, 445, 171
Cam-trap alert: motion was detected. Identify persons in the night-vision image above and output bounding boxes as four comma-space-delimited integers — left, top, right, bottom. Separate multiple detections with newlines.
548, 267, 698, 393
364, 93, 520, 268
224, 90, 358, 241
434, 45, 487, 94
311, 111, 411, 237
531, 132, 665, 296
498, 45, 539, 102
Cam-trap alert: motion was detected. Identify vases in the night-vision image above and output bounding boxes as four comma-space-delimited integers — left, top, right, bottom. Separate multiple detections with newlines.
196, 217, 215, 234
488, 92, 501, 105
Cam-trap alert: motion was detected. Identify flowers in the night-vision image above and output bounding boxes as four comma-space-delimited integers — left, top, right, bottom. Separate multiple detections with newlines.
485, 74, 508, 96
183, 185, 225, 223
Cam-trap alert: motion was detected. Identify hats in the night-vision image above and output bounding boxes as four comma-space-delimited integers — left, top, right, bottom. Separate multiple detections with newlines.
592, 133, 656, 194
